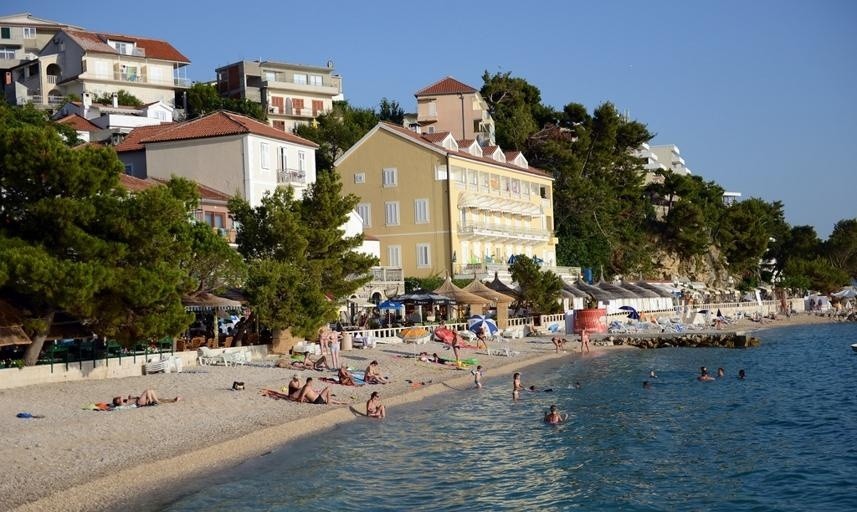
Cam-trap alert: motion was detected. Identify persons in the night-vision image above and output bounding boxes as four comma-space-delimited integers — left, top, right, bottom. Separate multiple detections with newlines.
716, 310, 722, 329
288, 374, 351, 405
367, 392, 385, 419
353, 309, 396, 328
475, 326, 487, 349
202, 315, 274, 347
686, 295, 694, 319
650, 370, 656, 377
338, 362, 356, 386
112, 388, 179, 406
552, 336, 566, 350
420, 330, 461, 367
699, 366, 745, 380
319, 328, 342, 370
580, 327, 589, 352
470, 367, 482, 388
704, 294, 712, 303
423, 310, 446, 325
364, 361, 388, 385
810, 298, 815, 310
817, 299, 823, 310
785, 304, 792, 318
278, 346, 331, 372
683, 291, 690, 305
512, 373, 552, 399
545, 406, 568, 424
529, 324, 538, 335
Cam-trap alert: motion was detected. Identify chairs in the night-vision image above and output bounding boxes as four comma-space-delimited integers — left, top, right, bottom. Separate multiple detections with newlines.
607, 316, 682, 335
196, 347, 248, 368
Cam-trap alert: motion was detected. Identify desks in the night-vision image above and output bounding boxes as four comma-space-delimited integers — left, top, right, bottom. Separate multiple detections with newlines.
291, 344, 320, 354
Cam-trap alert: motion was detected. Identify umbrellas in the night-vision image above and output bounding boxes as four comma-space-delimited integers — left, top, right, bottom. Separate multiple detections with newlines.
377, 270, 676, 329
830, 288, 857, 298
